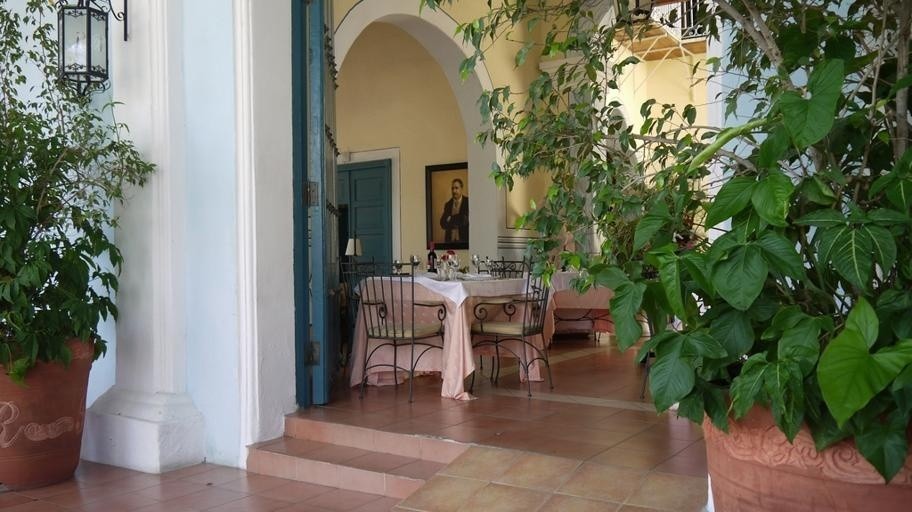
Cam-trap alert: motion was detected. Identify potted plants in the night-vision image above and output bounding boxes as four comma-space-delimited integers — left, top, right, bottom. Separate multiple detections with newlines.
0, 1, 154, 495
424, 2, 911, 512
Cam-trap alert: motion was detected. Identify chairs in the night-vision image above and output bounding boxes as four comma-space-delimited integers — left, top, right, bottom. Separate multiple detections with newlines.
340, 254, 556, 403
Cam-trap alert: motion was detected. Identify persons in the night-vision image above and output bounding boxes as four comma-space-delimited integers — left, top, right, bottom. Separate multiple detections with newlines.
440, 178, 469, 244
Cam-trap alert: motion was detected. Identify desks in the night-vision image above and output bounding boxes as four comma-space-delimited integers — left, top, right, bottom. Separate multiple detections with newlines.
548, 270, 649, 346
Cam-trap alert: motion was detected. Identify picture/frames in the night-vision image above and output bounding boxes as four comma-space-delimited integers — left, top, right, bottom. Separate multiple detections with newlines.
425, 162, 472, 251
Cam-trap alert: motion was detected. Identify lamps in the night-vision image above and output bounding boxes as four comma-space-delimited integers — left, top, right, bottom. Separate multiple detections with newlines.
56, 0, 127, 90
345, 238, 376, 269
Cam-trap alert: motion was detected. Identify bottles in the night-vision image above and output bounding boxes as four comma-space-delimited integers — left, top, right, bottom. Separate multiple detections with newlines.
427, 241, 438, 273
440, 256, 449, 281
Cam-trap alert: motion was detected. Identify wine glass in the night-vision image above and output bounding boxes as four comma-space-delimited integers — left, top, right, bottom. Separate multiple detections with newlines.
448, 255, 459, 282
472, 253, 480, 274
